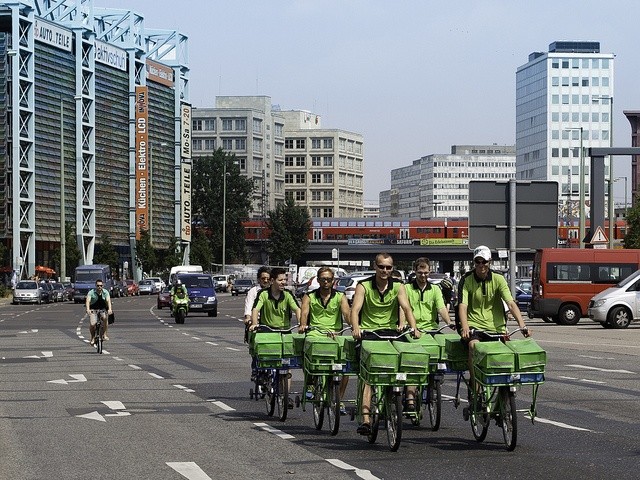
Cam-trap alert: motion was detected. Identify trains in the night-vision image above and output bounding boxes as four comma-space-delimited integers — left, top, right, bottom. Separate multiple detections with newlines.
190, 215, 632, 240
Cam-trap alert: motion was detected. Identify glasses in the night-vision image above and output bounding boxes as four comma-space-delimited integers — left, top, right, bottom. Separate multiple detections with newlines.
319, 278, 332, 282
475, 259, 488, 264
97, 285, 102, 287
376, 264, 394, 270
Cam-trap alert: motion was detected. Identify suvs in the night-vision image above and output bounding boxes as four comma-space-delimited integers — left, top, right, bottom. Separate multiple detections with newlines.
334, 275, 376, 307
146, 277, 166, 295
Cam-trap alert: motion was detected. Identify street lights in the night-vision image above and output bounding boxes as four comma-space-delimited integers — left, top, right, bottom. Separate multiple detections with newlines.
619, 174, 629, 237
564, 125, 585, 250
590, 95, 616, 250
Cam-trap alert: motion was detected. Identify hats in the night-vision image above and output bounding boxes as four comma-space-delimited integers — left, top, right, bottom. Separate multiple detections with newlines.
473, 246, 491, 260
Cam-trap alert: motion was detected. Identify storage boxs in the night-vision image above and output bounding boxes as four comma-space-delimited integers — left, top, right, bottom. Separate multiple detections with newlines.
361, 340, 399, 386
435, 332, 463, 361
336, 335, 361, 361
303, 337, 340, 376
393, 340, 430, 385
250, 331, 284, 368
445, 339, 469, 373
507, 338, 547, 385
342, 339, 363, 373
283, 333, 309, 356
406, 332, 441, 362
292, 337, 308, 358
474, 340, 516, 385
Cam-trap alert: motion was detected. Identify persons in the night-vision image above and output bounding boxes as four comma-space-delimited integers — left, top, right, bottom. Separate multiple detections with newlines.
244, 267, 274, 380
86, 279, 111, 346
351, 254, 421, 435
170, 279, 191, 317
301, 267, 351, 415
441, 287, 452, 312
458, 245, 532, 409
249, 267, 301, 410
399, 257, 455, 408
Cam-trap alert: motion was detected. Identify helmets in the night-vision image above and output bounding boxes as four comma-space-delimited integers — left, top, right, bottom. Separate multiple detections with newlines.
175, 280, 182, 285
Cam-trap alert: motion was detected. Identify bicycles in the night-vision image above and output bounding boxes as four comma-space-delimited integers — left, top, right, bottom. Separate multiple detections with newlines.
247, 323, 301, 422
448, 325, 546, 451
403, 324, 460, 430
87, 309, 108, 354
344, 326, 429, 453
290, 324, 358, 435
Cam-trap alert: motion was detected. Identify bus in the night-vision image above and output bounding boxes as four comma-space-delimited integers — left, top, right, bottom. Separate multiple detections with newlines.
74, 265, 111, 304
530, 249, 640, 325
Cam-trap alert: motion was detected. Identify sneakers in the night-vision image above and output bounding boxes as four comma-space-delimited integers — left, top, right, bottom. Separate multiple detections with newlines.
405, 405, 415, 412
91, 337, 95, 345
305, 384, 315, 400
102, 336, 109, 341
250, 371, 257, 380
357, 422, 372, 433
339, 403, 346, 415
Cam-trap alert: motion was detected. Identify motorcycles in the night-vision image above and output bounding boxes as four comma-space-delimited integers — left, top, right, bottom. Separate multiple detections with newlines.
169, 292, 190, 324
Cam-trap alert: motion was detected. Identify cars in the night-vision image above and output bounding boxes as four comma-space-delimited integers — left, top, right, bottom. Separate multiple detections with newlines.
231, 279, 255, 297
125, 280, 141, 296
55, 283, 66, 302
138, 280, 156, 296
33, 283, 52, 303
13, 280, 41, 304
62, 285, 74, 301
46, 283, 58, 303
158, 287, 173, 309
516, 279, 532, 311
110, 279, 128, 298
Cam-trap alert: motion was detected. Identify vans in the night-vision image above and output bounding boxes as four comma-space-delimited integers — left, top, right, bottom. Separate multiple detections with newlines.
349, 271, 378, 276
588, 268, 640, 329
169, 265, 204, 287
174, 271, 218, 317
212, 275, 229, 293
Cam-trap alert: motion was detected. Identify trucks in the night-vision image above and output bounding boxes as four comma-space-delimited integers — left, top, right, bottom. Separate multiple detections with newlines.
296, 267, 347, 300
